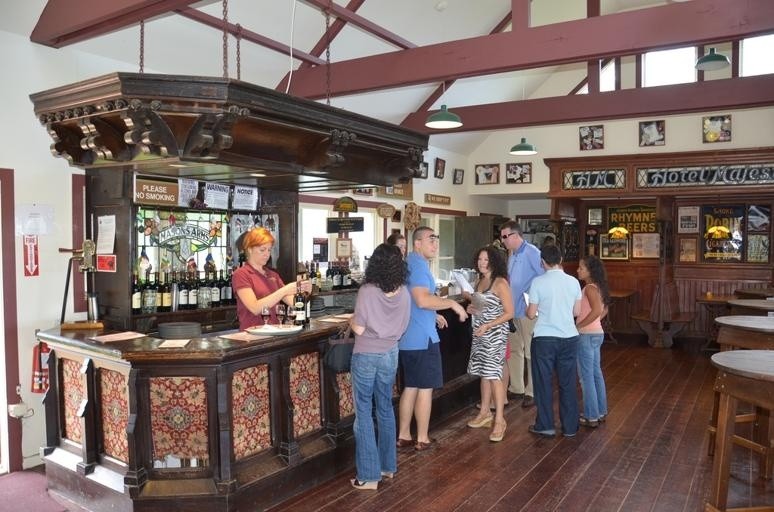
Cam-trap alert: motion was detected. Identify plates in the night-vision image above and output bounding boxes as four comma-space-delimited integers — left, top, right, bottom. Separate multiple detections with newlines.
157, 322, 200, 339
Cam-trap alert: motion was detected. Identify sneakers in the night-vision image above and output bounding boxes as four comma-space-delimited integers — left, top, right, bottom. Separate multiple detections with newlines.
522, 395, 536, 408
578, 412, 607, 427
475, 398, 510, 412
414, 437, 442, 451
396, 438, 417, 448
528, 424, 556, 439
561, 431, 577, 437
508, 392, 525, 401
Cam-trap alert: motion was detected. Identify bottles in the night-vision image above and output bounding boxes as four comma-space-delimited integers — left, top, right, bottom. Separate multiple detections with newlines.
293, 276, 306, 327
302, 274, 312, 323
131, 245, 238, 314
587, 234, 595, 256
85, 293, 98, 322
299, 260, 352, 290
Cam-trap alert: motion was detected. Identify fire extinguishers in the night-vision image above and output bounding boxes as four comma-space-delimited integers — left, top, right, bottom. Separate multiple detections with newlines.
31, 342, 50, 393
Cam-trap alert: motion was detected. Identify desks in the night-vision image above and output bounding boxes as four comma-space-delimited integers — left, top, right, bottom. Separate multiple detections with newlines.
599, 288, 638, 345
694, 286, 773, 511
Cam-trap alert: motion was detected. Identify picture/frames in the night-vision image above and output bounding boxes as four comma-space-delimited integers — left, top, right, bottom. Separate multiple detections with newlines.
578, 125, 603, 151
701, 113, 732, 144
637, 121, 666, 147
352, 151, 532, 195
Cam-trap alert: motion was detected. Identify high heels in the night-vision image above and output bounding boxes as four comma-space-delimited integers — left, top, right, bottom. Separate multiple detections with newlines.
381, 471, 394, 479
489, 418, 508, 442
349, 478, 379, 490
467, 414, 494, 428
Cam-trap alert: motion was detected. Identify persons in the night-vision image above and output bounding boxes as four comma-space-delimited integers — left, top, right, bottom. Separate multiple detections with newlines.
498, 221, 545, 407
526, 245, 582, 437
399, 227, 467, 451
462, 244, 516, 443
348, 244, 412, 491
232, 228, 309, 332
388, 233, 406, 255
542, 236, 554, 249
576, 254, 614, 428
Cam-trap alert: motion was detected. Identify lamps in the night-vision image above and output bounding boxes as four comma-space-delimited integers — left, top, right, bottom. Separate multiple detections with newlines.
607, 195, 629, 240
424, 1, 463, 130
703, 194, 733, 241
509, 69, 536, 156
693, 42, 732, 72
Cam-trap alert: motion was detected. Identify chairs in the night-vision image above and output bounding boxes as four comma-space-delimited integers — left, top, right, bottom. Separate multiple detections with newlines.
629, 281, 660, 346
664, 279, 698, 348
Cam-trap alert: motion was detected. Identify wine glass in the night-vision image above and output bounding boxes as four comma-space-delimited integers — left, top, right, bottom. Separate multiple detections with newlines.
434, 283, 442, 298
261, 304, 299, 331
